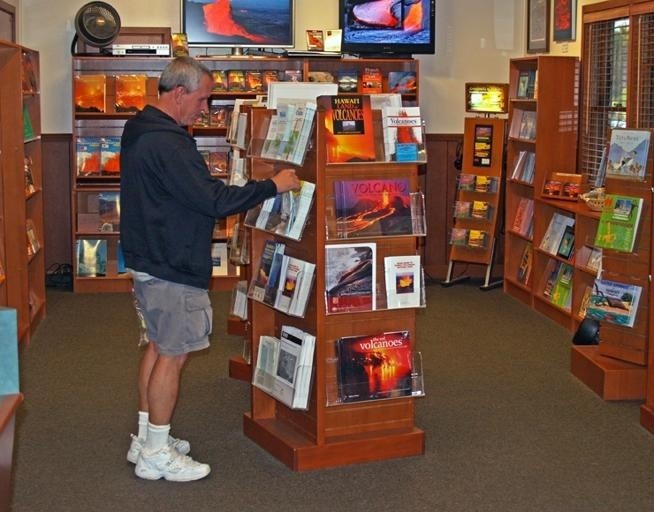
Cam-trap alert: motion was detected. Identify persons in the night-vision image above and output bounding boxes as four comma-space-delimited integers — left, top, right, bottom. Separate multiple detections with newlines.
122, 56, 302, 477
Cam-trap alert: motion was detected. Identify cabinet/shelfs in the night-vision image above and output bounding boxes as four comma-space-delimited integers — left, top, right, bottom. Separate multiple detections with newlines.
228, 105, 425, 472
505, 55, 575, 329
571, 125, 654, 401
72, 55, 419, 293
0, 39, 47, 347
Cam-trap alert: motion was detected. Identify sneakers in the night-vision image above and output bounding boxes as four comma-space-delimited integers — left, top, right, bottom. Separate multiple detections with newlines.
126, 433, 191, 462
134, 446, 211, 483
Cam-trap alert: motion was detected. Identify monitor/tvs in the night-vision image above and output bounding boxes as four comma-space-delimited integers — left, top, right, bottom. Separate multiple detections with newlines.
339, 0, 435, 58
180, 0, 295, 58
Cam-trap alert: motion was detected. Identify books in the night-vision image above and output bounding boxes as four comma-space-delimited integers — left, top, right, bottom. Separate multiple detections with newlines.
334, 180, 414, 238
22, 152, 40, 194
382, 106, 427, 162
26, 220, 45, 258
578, 241, 601, 276
97, 190, 120, 235
78, 138, 101, 180
335, 68, 361, 93
384, 256, 423, 309
450, 227, 489, 250
325, 245, 375, 314
172, 33, 189, 57
71, 72, 107, 113
512, 194, 536, 235
506, 107, 545, 143
307, 30, 344, 53
252, 323, 317, 412
595, 194, 644, 253
205, 150, 236, 176
97, 136, 124, 180
211, 243, 228, 276
242, 179, 316, 243
336, 330, 414, 403
360, 67, 384, 98
515, 68, 542, 97
545, 261, 577, 312
452, 199, 491, 221
248, 240, 315, 318
517, 243, 535, 283
604, 126, 650, 182
389, 68, 420, 101
207, 69, 305, 92
19, 47, 39, 93
317, 96, 376, 164
256, 102, 314, 162
472, 125, 494, 167
116, 73, 142, 120
457, 173, 497, 194
537, 211, 577, 260
588, 278, 643, 329
18, 99, 40, 140
78, 239, 106, 278
507, 149, 538, 182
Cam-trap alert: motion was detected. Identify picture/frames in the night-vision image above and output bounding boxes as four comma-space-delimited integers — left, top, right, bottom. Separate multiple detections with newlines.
526, 0, 550, 54
554, 0, 576, 42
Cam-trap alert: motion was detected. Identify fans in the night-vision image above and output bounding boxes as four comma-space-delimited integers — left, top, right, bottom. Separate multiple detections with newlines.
70, 1, 121, 58
